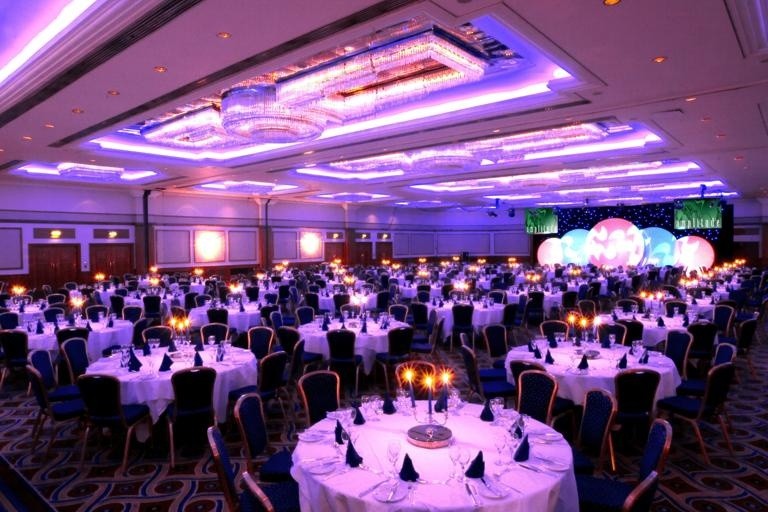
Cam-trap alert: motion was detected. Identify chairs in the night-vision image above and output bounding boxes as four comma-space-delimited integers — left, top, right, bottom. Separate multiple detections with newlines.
575, 418, 674, 511
276, 259, 767, 432
654, 362, 738, 460
231, 390, 296, 481
204, 422, 301, 511
1, 258, 284, 466
573, 386, 617, 470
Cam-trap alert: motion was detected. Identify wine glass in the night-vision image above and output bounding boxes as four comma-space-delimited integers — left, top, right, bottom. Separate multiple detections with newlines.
609, 302, 684, 324
22, 311, 117, 337
202, 294, 251, 313
429, 292, 489, 308
334, 387, 571, 507
313, 306, 396, 335
103, 333, 236, 375
535, 329, 645, 371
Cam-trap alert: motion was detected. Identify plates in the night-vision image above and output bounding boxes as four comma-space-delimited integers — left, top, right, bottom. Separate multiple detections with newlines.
297, 432, 323, 442
304, 459, 334, 476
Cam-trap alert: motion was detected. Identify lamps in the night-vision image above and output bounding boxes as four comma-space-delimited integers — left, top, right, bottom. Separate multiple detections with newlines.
330, 117, 744, 211
273, 27, 487, 123
221, 84, 326, 145
142, 104, 248, 151
